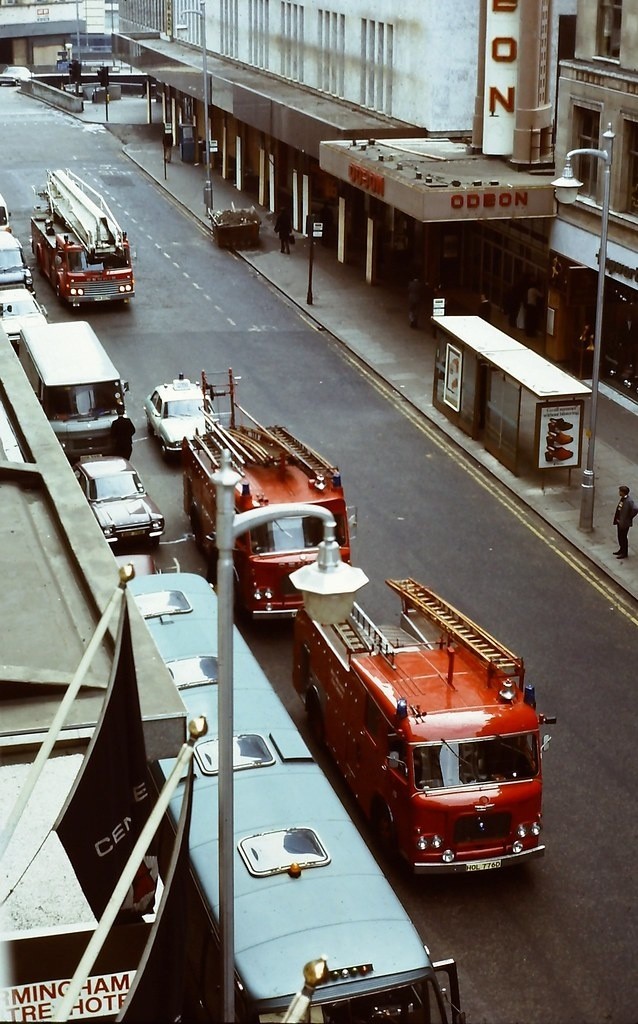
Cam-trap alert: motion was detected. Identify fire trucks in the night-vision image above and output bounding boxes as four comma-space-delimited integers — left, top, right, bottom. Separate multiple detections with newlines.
291, 576, 557, 876
29, 167, 136, 308
181, 366, 360, 621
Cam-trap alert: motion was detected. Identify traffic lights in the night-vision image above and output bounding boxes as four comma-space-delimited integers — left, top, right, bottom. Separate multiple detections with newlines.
95, 66, 108, 87
68, 60, 81, 82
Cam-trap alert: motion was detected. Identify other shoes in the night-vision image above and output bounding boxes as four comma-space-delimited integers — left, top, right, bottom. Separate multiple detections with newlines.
286, 252, 290, 254
612, 550, 622, 555
616, 554, 628, 559
281, 251, 285, 253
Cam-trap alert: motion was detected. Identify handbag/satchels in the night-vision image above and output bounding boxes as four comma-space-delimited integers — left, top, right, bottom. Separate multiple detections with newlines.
289, 233, 295, 244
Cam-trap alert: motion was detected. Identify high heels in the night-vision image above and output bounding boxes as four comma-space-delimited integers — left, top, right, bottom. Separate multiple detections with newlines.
545, 430, 574, 447
548, 417, 574, 432
544, 444, 573, 462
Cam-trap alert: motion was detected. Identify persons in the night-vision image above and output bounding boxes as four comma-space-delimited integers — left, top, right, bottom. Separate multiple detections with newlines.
407, 276, 422, 328
507, 272, 545, 337
274, 207, 292, 254
141, 80, 149, 98
613, 485, 638, 559
479, 293, 491, 321
163, 134, 173, 163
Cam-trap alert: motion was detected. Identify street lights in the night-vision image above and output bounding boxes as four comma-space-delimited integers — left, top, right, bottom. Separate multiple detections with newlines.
176, 1, 214, 218
211, 449, 369, 1022
550, 122, 617, 536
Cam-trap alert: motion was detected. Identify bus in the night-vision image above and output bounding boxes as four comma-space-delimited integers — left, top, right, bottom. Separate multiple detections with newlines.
118, 570, 465, 1022
19, 320, 130, 464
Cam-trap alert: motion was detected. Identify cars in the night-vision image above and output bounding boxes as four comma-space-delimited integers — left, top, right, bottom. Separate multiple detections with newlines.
0, 230, 37, 298
142, 372, 222, 459
0, 193, 12, 234
71, 456, 167, 552
0, 289, 49, 345
0, 65, 31, 86
